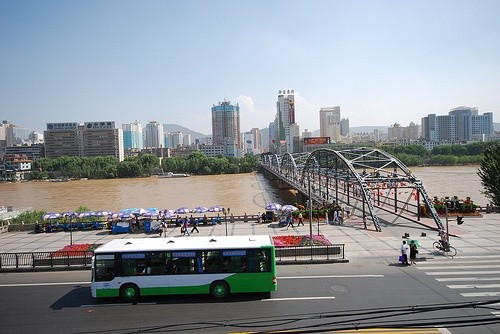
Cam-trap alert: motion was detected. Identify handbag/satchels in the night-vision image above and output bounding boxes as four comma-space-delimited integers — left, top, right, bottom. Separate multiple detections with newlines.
399, 256, 404, 261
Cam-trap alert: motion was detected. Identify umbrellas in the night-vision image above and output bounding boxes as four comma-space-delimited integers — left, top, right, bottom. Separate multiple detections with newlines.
281, 205, 300, 214
407, 239, 420, 250
40, 204, 227, 232
264, 203, 282, 212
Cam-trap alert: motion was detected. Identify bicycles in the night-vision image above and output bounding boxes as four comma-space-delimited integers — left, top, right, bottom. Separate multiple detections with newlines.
432, 240, 457, 258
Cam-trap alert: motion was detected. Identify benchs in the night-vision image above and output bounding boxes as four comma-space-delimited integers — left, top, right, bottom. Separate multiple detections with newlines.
48, 217, 221, 233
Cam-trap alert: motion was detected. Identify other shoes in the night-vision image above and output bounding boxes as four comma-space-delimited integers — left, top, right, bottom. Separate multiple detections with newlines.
402, 263, 405, 264
407, 264, 410, 265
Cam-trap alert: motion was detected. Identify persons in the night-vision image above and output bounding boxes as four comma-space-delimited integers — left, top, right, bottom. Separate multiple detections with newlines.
401, 241, 409, 265
323, 210, 329, 225
338, 209, 343, 226
134, 257, 263, 274
362, 215, 368, 230
257, 211, 268, 223
332, 211, 339, 224
41, 222, 59, 232
298, 211, 304, 227
440, 231, 448, 252
130, 213, 222, 236
286, 215, 296, 231
409, 240, 418, 266
345, 204, 351, 218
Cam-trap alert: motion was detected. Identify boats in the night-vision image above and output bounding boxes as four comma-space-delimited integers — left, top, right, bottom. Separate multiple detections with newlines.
157, 171, 189, 178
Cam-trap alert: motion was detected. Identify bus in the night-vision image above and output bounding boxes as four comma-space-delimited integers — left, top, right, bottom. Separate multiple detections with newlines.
89, 234, 278, 302
